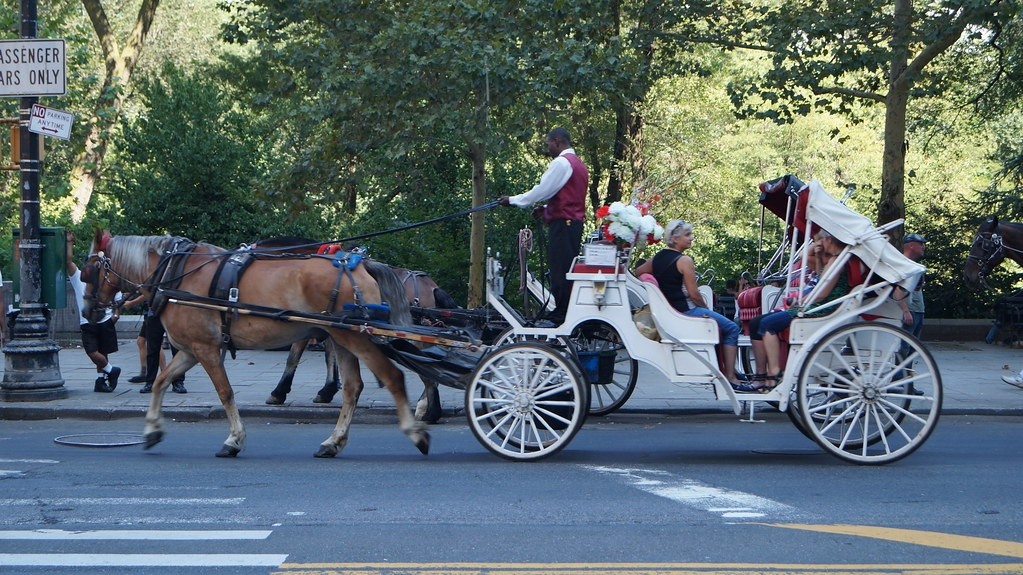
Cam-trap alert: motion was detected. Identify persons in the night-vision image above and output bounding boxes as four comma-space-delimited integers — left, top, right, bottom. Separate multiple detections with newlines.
1002, 370, 1023, 388
894, 233, 924, 395
750, 232, 849, 394
123, 294, 187, 393
497, 127, 588, 327
0, 270, 6, 341
635, 220, 750, 392
66, 229, 123, 392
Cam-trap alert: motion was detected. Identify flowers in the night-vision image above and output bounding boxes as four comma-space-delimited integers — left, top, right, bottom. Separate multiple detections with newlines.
595, 172, 682, 251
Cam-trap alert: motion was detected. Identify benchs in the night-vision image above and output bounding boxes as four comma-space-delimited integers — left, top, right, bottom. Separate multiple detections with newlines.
566, 255, 627, 282
639, 273, 724, 344
737, 256, 863, 345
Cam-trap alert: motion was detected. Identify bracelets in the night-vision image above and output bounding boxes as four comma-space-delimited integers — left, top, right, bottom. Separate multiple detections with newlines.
114, 314, 120, 319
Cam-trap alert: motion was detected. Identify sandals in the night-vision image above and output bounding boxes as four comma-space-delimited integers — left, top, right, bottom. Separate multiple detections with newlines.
744, 373, 767, 391
758, 371, 784, 394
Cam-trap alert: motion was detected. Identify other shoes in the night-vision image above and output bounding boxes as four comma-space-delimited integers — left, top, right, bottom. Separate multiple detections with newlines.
737, 373, 747, 381
537, 320, 556, 328
729, 382, 754, 394
128, 376, 146, 382
542, 311, 565, 319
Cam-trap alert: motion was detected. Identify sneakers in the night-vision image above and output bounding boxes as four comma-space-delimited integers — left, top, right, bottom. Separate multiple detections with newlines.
1001, 370, 1023, 389
172, 381, 187, 393
912, 388, 924, 395
139, 383, 153, 393
94, 377, 112, 392
106, 367, 121, 391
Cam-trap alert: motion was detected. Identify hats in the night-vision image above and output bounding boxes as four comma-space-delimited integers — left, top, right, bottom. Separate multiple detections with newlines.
902, 233, 928, 243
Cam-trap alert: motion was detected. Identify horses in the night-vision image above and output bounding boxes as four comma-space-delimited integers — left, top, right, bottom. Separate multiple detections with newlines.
82, 224, 460, 459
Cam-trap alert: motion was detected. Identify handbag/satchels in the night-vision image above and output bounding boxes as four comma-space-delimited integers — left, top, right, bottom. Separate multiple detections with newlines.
681, 281, 708, 310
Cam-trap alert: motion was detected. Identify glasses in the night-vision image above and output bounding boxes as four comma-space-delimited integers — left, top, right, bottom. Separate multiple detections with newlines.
671, 220, 686, 235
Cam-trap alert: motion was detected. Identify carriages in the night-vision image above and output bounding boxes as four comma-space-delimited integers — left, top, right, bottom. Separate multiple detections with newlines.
82, 172, 944, 465
